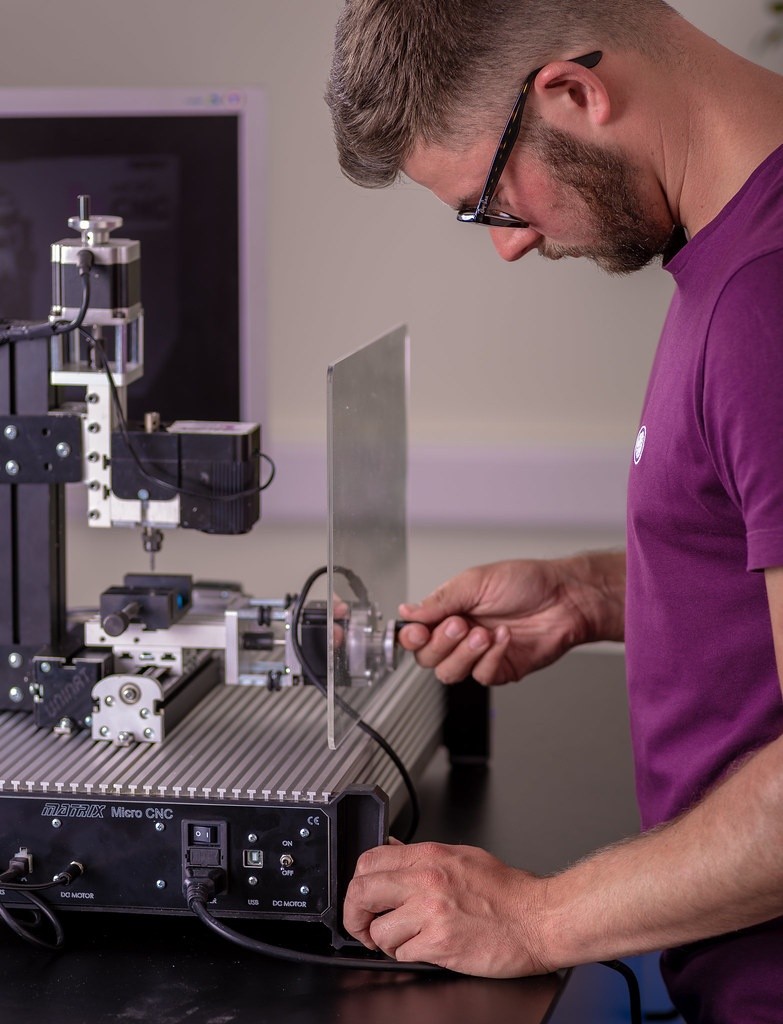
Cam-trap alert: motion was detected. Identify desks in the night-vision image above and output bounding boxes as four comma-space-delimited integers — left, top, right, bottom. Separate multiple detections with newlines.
0, 912, 575, 1024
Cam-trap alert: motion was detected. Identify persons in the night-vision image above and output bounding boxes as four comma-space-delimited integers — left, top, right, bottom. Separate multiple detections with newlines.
324, 0, 783, 1024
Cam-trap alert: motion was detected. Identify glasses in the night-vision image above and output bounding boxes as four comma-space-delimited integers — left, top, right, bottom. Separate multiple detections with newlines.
457, 49, 604, 229
